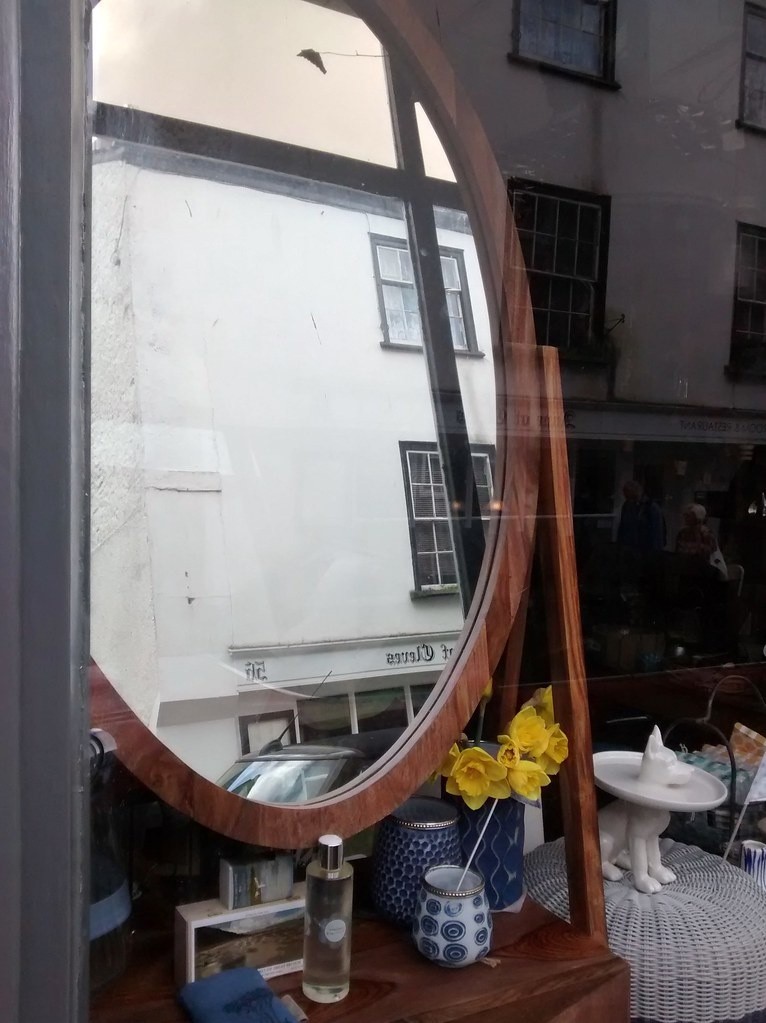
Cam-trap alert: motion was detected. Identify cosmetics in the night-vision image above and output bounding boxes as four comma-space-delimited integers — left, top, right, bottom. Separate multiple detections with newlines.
300, 836, 354, 1003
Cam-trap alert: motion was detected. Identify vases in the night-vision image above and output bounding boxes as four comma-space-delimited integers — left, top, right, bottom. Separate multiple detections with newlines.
438, 742, 526, 910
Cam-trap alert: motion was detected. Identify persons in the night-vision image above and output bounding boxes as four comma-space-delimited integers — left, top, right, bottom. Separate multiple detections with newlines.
615, 481, 667, 559
581, 506, 741, 662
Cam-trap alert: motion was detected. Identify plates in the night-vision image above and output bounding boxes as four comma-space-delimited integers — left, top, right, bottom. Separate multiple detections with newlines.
592, 749, 734, 808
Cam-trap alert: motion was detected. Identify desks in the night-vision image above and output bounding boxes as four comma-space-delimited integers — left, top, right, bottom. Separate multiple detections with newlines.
88, 847, 630, 1023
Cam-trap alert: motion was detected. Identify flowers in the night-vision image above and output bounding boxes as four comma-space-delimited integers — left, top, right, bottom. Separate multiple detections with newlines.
427, 677, 569, 811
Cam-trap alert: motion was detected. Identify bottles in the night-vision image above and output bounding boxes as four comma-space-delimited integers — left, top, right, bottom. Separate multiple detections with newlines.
302, 835, 353, 1004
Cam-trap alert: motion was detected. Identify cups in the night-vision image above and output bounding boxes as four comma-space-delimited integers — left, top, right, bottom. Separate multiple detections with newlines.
372, 796, 460, 923
414, 865, 493, 968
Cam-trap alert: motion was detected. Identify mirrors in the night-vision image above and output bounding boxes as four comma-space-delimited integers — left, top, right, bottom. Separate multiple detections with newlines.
84, 0, 531, 852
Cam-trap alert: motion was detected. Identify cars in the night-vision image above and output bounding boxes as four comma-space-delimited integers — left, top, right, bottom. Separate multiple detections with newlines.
212, 726, 409, 803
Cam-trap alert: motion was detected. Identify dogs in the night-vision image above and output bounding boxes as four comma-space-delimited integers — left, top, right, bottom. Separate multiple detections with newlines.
597, 724, 691, 894
